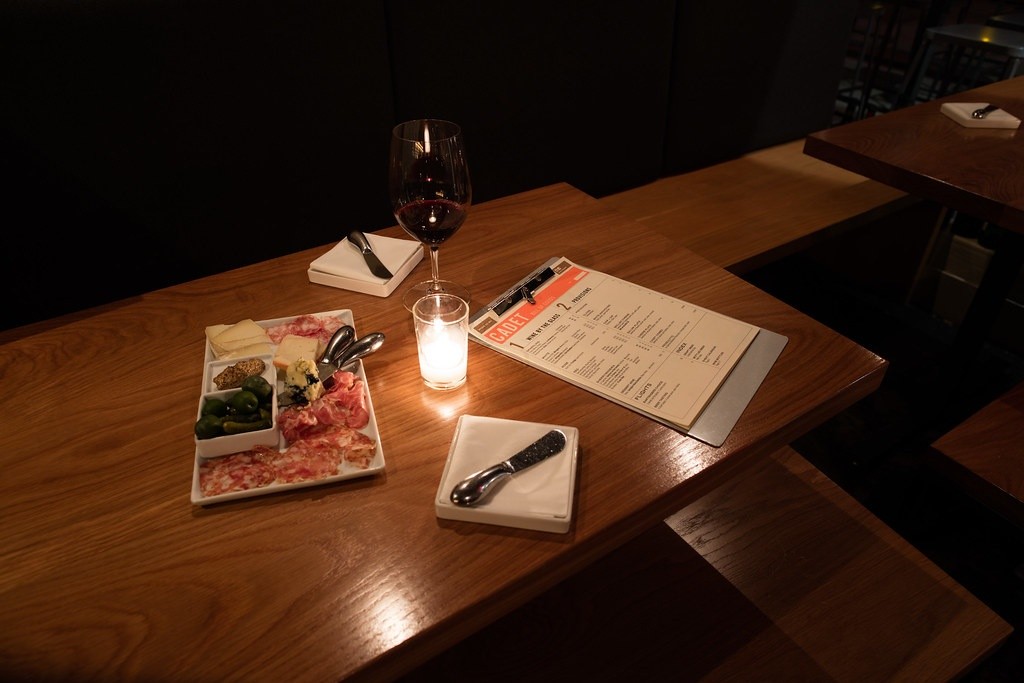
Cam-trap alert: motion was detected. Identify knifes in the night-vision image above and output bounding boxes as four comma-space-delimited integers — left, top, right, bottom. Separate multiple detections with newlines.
450, 429, 569, 506
972, 103, 1000, 119
277, 326, 384, 407
347, 231, 393, 279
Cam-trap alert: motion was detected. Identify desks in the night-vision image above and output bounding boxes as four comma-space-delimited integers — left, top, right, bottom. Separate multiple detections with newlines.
804, 73, 1024, 325
0, 182, 889, 683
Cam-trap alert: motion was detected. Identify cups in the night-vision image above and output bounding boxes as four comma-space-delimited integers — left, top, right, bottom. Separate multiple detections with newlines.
412, 294, 469, 392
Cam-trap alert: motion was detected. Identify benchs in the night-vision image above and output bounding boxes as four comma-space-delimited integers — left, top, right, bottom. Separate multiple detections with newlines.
928, 379, 1024, 530
599, 135, 929, 278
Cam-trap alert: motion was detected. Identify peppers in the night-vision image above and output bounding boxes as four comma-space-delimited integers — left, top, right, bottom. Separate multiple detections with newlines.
193, 374, 272, 439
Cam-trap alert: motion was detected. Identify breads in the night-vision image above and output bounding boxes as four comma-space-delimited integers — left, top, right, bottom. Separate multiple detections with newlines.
204, 319, 320, 371
213, 358, 265, 390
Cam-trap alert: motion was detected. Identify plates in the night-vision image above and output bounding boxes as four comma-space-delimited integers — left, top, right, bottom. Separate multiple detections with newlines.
307, 231, 425, 299
939, 102, 1021, 129
194, 352, 281, 458
436, 414, 580, 534
188, 307, 386, 506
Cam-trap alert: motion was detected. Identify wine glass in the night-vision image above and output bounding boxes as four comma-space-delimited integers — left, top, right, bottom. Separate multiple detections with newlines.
390, 117, 473, 317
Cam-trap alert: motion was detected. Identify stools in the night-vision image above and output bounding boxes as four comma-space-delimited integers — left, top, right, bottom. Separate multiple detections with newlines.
827, 0, 1024, 128
